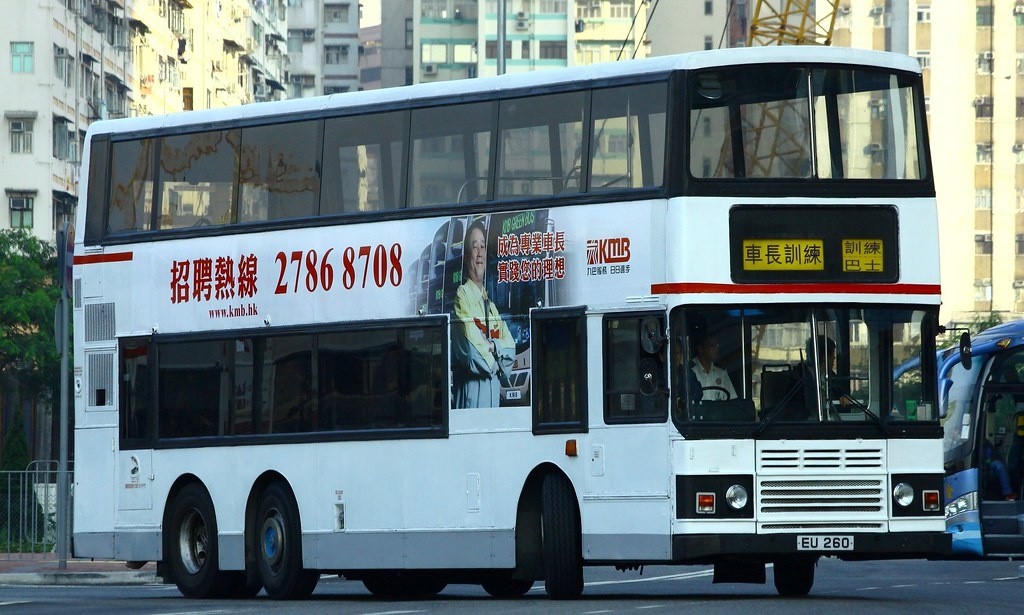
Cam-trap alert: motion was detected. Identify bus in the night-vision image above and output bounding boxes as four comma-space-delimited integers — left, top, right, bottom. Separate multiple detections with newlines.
69, 43, 952, 599
892, 320, 1024, 562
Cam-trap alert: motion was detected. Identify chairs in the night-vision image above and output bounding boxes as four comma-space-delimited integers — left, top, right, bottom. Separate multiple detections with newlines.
759, 363, 793, 413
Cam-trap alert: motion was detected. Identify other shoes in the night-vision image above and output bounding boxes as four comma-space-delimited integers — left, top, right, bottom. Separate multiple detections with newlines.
1004, 495, 1015, 502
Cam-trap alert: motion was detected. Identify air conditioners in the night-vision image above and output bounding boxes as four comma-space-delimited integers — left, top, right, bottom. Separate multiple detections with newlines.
91, 0, 98, 5
841, 5, 1023, 289
9, 121, 24, 132
340, 46, 347, 52
467, 67, 475, 77
591, 0, 601, 7
292, 76, 302, 83
576, 19, 583, 32
55, 47, 68, 57
304, 30, 313, 38
423, 64, 436, 75
9, 197, 27, 210
284, 70, 290, 82
516, 11, 529, 20
515, 21, 529, 30
253, 75, 261, 84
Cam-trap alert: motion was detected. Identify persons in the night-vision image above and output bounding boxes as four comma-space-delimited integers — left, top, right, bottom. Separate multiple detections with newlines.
986, 439, 1018, 501
783, 334, 849, 413
639, 337, 703, 416
451, 220, 516, 409
689, 332, 738, 401
